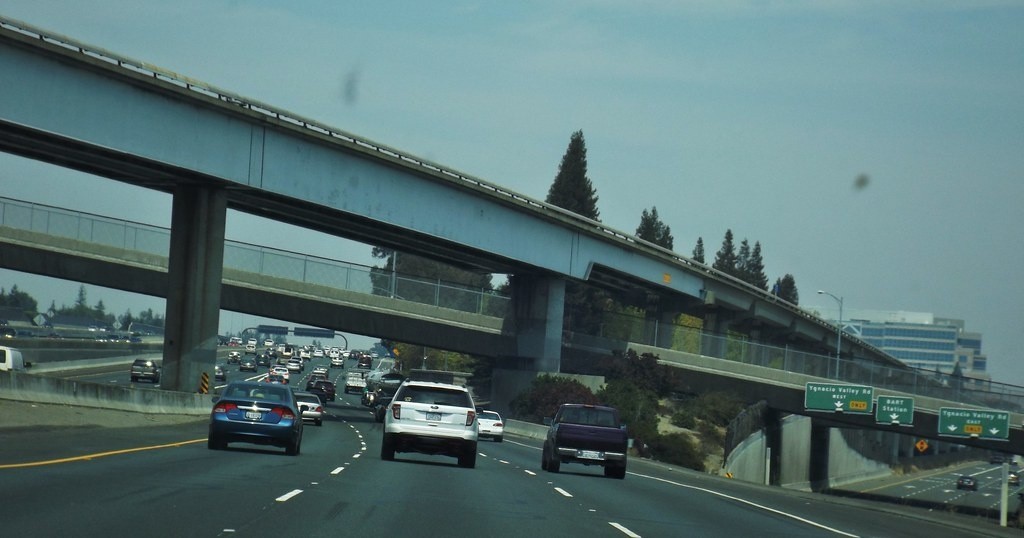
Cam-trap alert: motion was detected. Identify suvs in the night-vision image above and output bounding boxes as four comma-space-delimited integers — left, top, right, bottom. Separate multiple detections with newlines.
380, 377, 478, 468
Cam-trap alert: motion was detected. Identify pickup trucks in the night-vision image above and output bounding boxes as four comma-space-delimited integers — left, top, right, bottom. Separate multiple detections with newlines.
542, 403, 628, 480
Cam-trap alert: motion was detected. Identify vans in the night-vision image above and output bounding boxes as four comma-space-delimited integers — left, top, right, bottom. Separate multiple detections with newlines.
0, 345, 33, 374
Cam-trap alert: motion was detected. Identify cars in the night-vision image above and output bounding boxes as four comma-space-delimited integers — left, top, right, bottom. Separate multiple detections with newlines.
1007, 471, 1020, 485
207, 381, 309, 456
131, 357, 162, 383
990, 456, 1003, 464
1, 319, 151, 347
214, 336, 490, 426
475, 410, 504, 441
955, 476, 978, 490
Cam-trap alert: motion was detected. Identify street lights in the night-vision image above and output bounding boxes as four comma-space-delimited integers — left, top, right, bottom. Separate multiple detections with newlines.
817, 289, 844, 381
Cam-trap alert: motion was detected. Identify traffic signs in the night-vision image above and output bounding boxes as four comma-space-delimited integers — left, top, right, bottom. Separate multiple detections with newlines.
804, 382, 874, 415
938, 407, 1010, 442
875, 395, 915, 427
